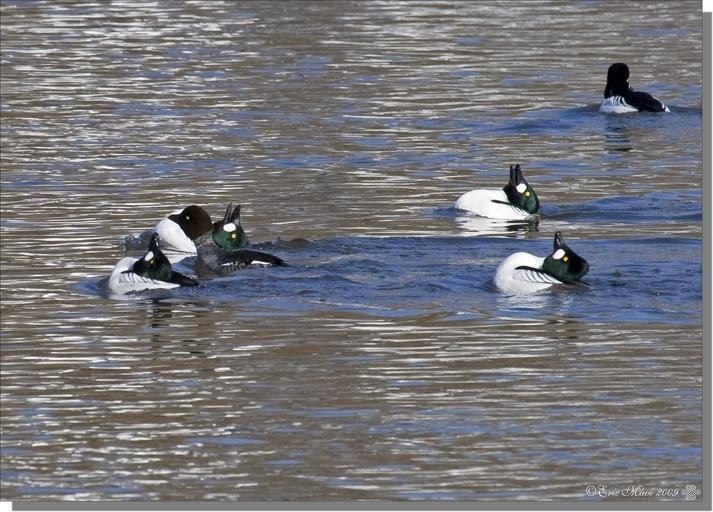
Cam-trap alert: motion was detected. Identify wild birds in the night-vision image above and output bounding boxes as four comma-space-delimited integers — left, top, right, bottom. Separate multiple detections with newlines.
453, 162, 541, 223
493, 231, 591, 298
597, 61, 671, 117
107, 203, 286, 299
